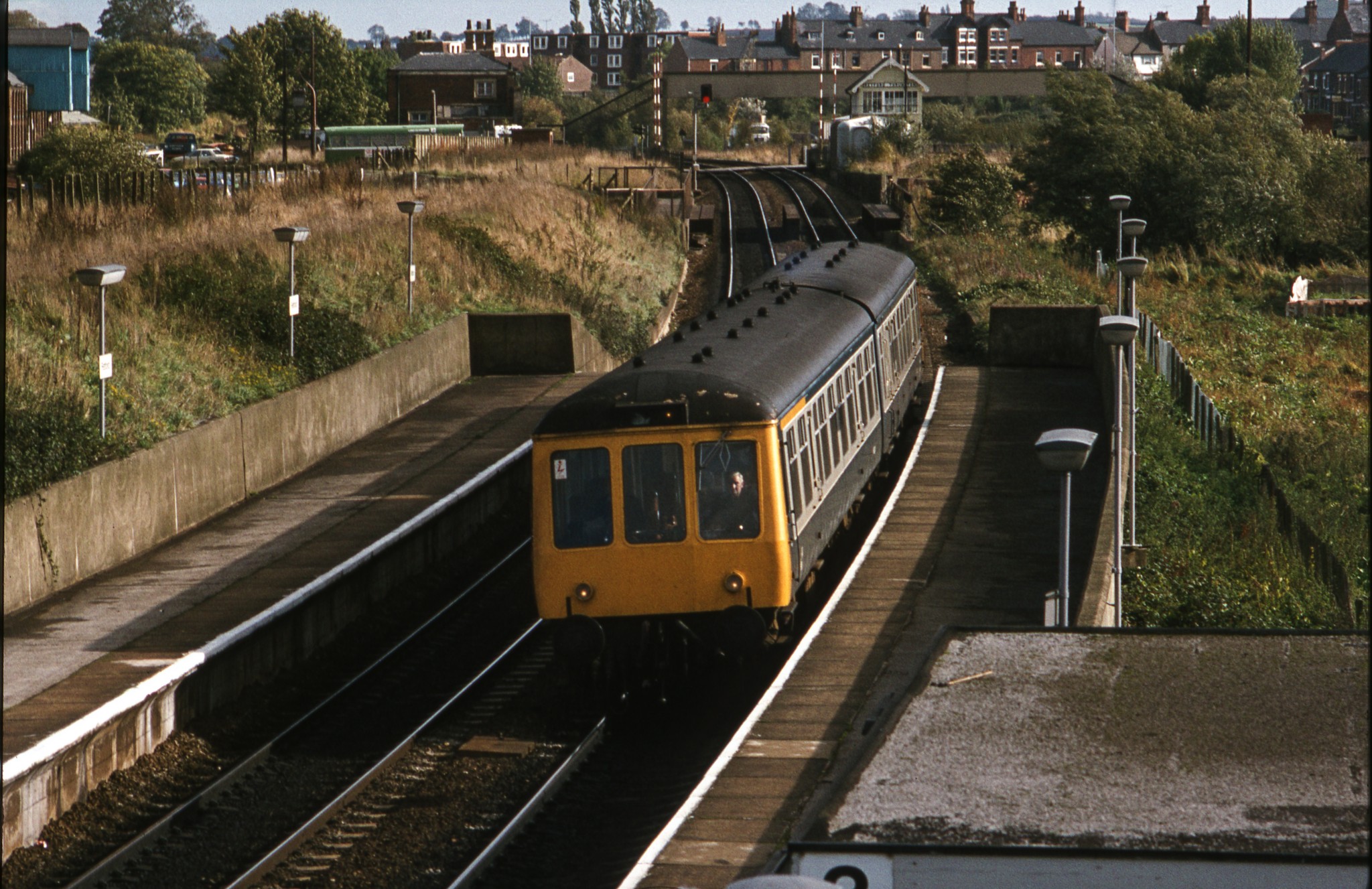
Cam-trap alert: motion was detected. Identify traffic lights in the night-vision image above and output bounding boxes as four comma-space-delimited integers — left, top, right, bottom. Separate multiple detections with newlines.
636, 124, 643, 135
632, 124, 638, 134
700, 84, 713, 103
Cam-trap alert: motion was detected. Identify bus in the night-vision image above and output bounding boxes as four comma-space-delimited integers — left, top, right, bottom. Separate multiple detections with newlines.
324, 122, 464, 167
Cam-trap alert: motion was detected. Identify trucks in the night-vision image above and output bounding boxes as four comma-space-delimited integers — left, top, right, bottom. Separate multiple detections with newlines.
726, 111, 773, 149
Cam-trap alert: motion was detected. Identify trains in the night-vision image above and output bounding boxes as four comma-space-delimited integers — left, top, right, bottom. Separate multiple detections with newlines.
530, 238, 925, 705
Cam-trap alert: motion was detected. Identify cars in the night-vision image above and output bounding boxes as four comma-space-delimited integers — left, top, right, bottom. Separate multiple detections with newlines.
128, 132, 248, 195
267, 128, 326, 151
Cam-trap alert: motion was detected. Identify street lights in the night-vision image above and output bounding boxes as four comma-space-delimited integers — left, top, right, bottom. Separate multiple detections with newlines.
1033, 428, 1099, 629
397, 200, 425, 317
77, 262, 127, 440
1114, 255, 1151, 548
1097, 314, 1140, 629
1119, 218, 1149, 373
292, 76, 317, 168
1108, 194, 1132, 313
272, 226, 311, 364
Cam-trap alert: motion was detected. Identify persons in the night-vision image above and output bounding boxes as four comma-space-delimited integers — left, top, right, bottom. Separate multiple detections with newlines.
710, 471, 758, 531
